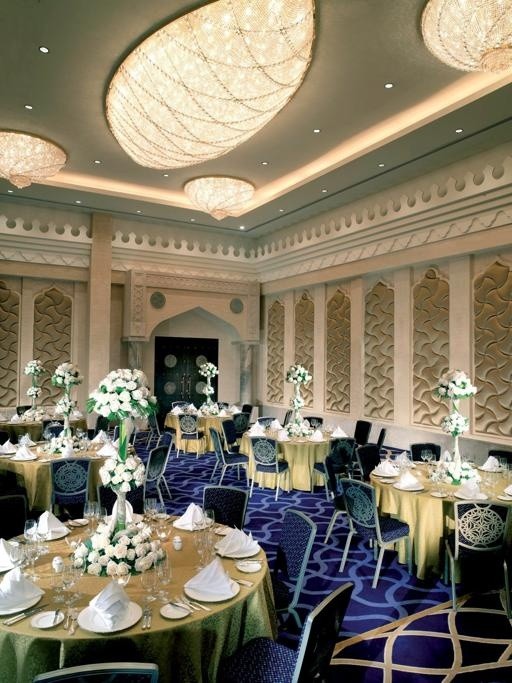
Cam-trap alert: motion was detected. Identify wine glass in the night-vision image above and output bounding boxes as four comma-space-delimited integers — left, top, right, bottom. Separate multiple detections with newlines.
84, 502, 99, 535
425, 450, 432, 470
192, 509, 215, 573
311, 418, 335, 438
497, 453, 512, 481
62, 558, 77, 606
158, 559, 172, 603
141, 564, 156, 602
75, 427, 99, 457
96, 507, 108, 533
49, 563, 67, 604
23, 520, 37, 540
25, 540, 41, 582
420, 450, 427, 468
143, 498, 156, 532
429, 455, 436, 472
146, 561, 161, 595
72, 554, 86, 601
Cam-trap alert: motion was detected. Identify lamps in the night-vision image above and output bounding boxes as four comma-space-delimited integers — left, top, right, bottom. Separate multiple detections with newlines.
420, 0, 512, 73
184, 175, 255, 221
105, 0, 316, 171
0, 127, 68, 189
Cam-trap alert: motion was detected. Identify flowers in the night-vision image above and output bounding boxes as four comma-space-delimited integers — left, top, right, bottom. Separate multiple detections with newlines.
202, 385, 214, 403
51, 363, 84, 397
24, 360, 45, 387
198, 362, 218, 385
86, 368, 159, 457
26, 387, 42, 409
286, 363, 310, 394
433, 369, 476, 412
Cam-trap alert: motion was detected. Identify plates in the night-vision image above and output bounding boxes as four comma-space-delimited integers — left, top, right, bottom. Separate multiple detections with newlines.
497, 495, 512, 501
235, 561, 261, 574
0, 595, 42, 616
214, 528, 233, 535
380, 479, 396, 484
371, 472, 398, 478
35, 531, 71, 541
392, 483, 424, 491
173, 520, 214, 531
214, 542, 261, 558
9, 457, 37, 460
453, 492, 488, 500
0, 453, 16, 455
183, 579, 240, 603
31, 611, 64, 629
103, 513, 143, 525
477, 466, 501, 473
152, 515, 170, 521
160, 603, 191, 620
430, 492, 448, 498
77, 601, 143, 634
69, 519, 88, 526
0, 559, 25, 572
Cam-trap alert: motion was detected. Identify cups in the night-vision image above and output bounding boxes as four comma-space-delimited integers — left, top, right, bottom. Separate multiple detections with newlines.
155, 520, 173, 542
112, 571, 131, 586
